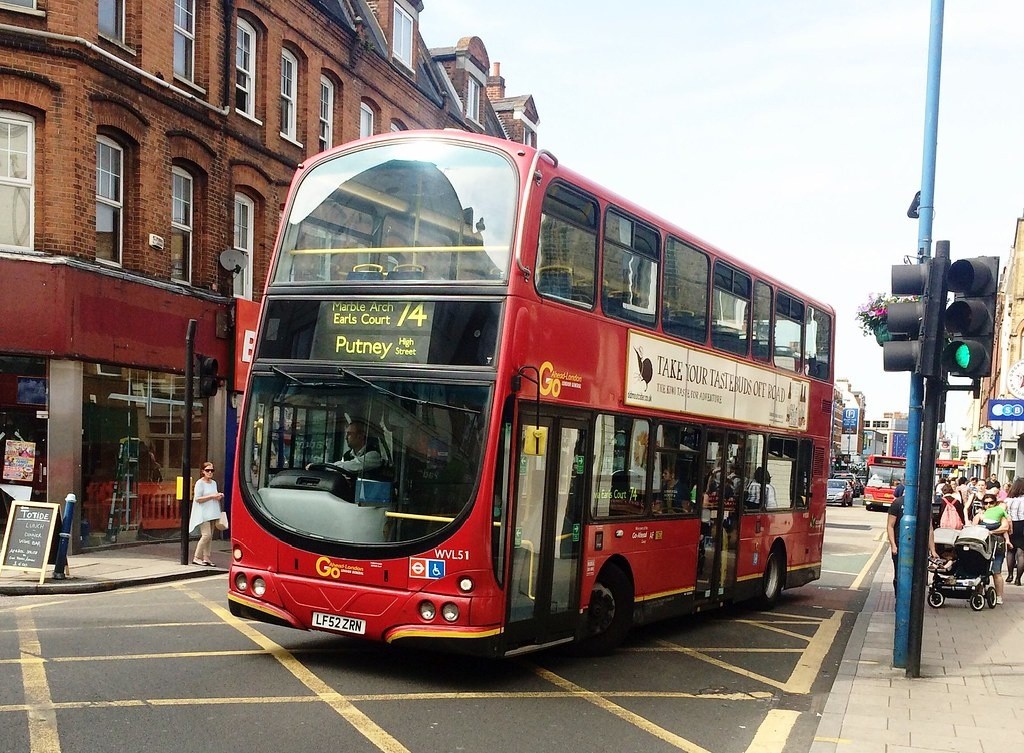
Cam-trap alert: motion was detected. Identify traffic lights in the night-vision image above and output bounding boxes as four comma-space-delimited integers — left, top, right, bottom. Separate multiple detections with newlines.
941, 256, 1001, 378
195, 353, 219, 399
883, 239, 950, 379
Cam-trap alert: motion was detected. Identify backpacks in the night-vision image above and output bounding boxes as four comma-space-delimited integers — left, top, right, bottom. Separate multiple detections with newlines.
939, 496, 963, 530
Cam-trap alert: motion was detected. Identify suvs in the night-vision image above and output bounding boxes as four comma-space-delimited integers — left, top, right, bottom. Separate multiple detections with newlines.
833, 473, 861, 498
137, 440, 164, 483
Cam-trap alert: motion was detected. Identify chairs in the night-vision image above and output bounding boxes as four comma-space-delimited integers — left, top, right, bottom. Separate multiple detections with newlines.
346, 264, 384, 279
383, 264, 425, 280
532, 263, 830, 385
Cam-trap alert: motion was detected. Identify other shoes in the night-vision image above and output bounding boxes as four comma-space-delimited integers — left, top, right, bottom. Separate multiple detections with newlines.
192, 559, 207, 566
202, 559, 217, 567
997, 595, 1003, 604
1014, 577, 1021, 586
1005, 576, 1013, 582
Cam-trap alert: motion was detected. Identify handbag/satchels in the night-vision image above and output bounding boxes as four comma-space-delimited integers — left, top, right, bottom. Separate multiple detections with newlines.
215, 497, 228, 531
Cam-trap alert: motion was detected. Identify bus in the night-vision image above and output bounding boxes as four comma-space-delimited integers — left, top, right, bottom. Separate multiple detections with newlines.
863, 454, 907, 512
226, 128, 837, 670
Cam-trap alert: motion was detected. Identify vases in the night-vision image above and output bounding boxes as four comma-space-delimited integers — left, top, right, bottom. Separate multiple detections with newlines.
872, 320, 907, 344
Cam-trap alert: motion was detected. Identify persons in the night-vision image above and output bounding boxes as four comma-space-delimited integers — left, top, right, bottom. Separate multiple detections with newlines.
746, 467, 776, 509
305, 421, 382, 481
705, 459, 721, 492
932, 474, 1024, 605
654, 464, 690, 509
887, 496, 939, 612
189, 461, 224, 566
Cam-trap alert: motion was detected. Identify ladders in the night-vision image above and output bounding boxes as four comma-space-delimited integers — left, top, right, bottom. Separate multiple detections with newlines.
106, 436, 141, 541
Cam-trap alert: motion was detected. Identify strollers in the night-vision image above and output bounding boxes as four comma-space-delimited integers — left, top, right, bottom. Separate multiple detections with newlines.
926, 525, 997, 611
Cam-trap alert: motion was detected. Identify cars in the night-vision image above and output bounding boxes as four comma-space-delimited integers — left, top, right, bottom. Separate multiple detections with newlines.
826, 479, 854, 507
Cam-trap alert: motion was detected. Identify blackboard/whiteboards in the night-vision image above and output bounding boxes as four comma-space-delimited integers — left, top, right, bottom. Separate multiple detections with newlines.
0, 501, 59, 572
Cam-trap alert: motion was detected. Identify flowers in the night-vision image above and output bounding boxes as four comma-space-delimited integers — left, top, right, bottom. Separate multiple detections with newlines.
852, 289, 923, 333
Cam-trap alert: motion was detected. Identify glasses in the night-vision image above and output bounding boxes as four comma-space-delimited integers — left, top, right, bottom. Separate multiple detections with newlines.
982, 500, 995, 505
204, 469, 215, 473
